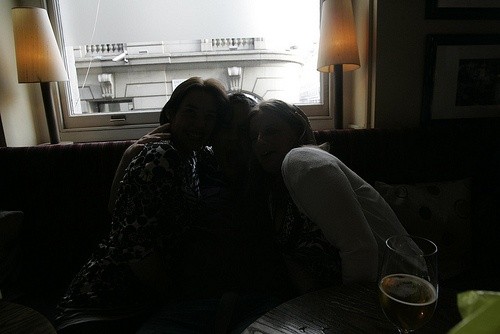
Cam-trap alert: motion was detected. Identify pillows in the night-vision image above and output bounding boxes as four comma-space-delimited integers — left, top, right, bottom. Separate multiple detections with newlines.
374, 180, 471, 280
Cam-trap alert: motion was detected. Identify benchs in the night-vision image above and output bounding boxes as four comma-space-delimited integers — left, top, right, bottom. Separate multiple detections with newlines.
0, 121, 500, 334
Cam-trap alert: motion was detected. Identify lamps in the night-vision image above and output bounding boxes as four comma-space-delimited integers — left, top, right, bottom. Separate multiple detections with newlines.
12, 6, 73, 146
317, 0, 362, 131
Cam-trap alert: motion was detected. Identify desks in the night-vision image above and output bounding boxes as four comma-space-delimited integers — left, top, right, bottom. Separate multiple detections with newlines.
241, 283, 460, 334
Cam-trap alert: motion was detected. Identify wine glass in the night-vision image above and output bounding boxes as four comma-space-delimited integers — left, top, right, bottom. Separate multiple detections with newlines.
377, 234, 438, 334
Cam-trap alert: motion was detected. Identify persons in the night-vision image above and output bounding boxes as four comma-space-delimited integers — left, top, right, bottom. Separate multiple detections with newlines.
107, 94, 292, 318
55, 78, 234, 334
247, 99, 428, 299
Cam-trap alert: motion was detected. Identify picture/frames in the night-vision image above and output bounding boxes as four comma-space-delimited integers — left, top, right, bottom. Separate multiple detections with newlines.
425, 0, 500, 21
419, 32, 499, 124
